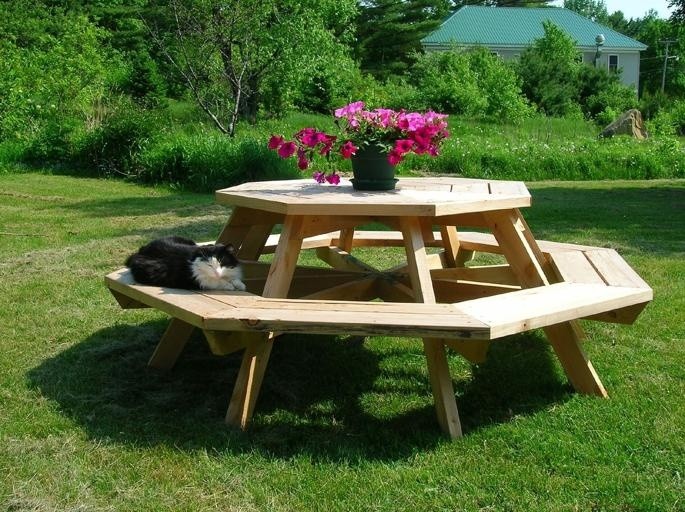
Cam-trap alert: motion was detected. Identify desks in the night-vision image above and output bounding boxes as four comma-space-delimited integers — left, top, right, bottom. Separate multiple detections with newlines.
146, 177, 609, 441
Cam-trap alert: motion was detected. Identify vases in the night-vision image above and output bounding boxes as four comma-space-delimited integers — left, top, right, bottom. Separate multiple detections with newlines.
349, 147, 399, 191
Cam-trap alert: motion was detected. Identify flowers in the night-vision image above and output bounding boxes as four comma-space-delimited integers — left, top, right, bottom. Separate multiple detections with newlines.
267, 101, 449, 185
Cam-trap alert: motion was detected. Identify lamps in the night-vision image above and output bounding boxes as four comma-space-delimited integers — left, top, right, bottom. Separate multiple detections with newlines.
596, 34, 606, 58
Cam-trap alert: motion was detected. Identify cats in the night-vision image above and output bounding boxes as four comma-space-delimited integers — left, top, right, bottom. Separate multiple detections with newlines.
122, 235, 246, 292
